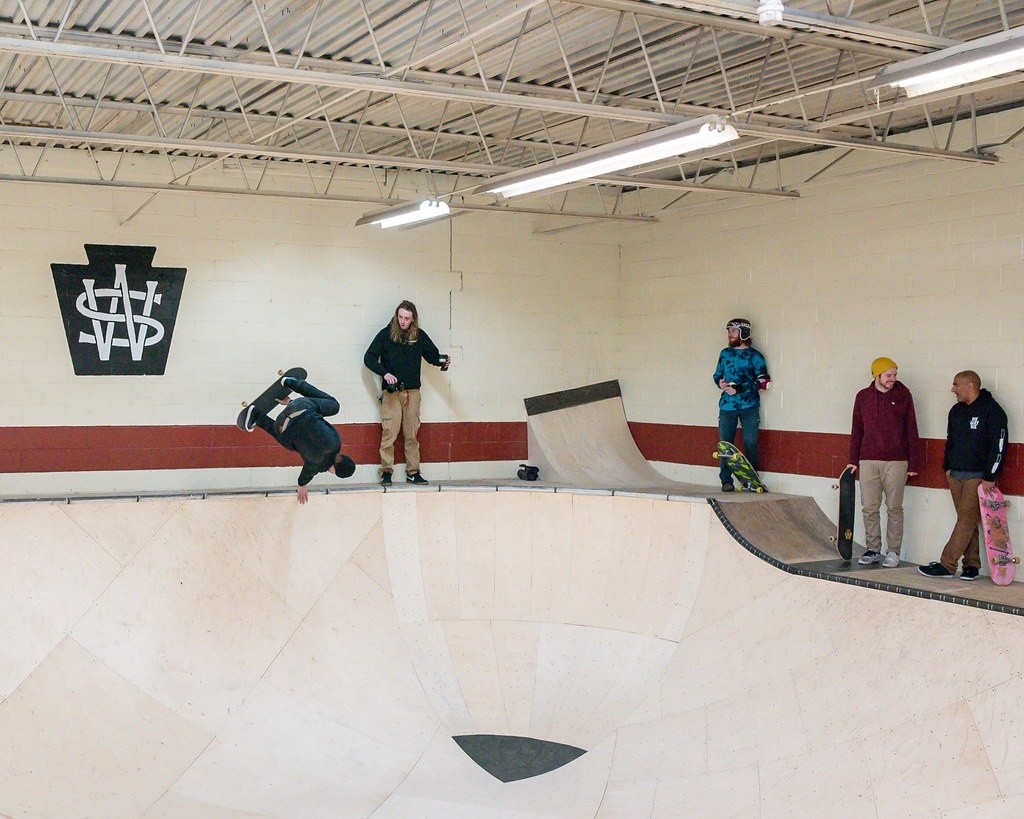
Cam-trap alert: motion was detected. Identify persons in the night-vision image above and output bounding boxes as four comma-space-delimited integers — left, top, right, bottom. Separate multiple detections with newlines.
916, 370, 1008, 581
362, 302, 452, 485
714, 318, 773, 494
846, 357, 924, 570
244, 374, 358, 505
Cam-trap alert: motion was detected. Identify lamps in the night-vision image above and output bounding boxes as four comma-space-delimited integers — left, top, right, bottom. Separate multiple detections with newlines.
468, 114, 741, 205
867, 25, 1024, 99
353, 196, 451, 229
756, 1, 784, 29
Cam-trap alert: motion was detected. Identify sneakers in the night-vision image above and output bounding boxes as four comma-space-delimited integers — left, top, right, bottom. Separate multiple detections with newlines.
721, 483, 734, 492
245, 404, 257, 433
281, 376, 297, 387
959, 564, 979, 581
917, 562, 955, 577
881, 551, 900, 567
380, 470, 392, 486
406, 472, 429, 485
857, 548, 882, 565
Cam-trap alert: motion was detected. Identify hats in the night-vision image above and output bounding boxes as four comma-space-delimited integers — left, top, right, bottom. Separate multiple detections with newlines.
726, 318, 752, 340
871, 357, 898, 379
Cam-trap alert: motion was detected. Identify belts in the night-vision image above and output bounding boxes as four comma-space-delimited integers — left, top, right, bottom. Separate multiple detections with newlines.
281, 409, 308, 433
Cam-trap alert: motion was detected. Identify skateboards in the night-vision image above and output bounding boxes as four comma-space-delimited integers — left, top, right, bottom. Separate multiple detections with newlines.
827, 466, 856, 561
712, 441, 768, 494
976, 482, 1020, 586
236, 367, 308, 431
